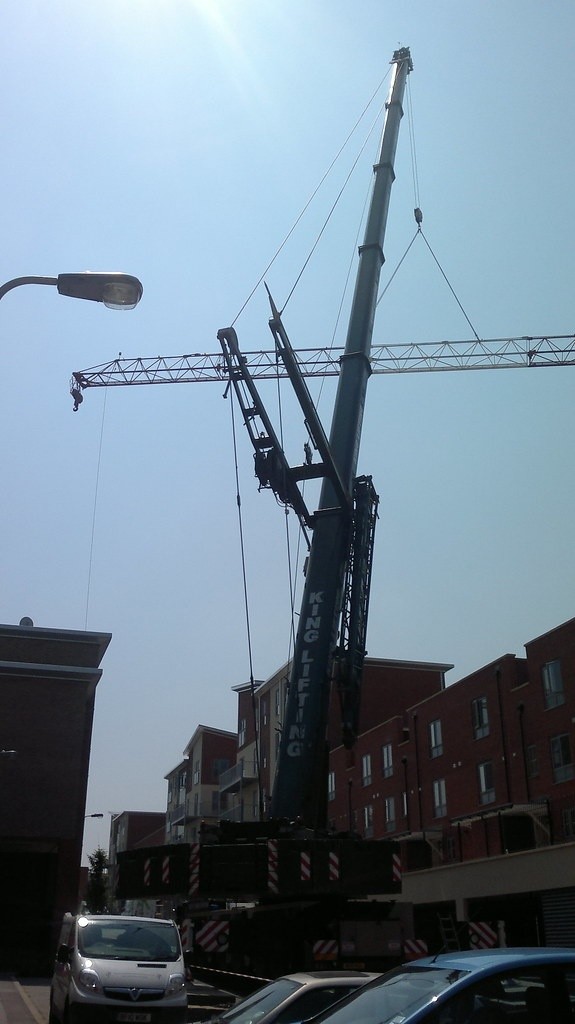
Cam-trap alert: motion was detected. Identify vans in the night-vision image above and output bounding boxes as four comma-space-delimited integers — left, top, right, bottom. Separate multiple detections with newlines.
49, 914, 189, 1024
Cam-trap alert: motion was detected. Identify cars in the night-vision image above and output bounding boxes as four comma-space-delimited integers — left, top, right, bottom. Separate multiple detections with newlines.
297, 949, 575, 1024
189, 970, 546, 1024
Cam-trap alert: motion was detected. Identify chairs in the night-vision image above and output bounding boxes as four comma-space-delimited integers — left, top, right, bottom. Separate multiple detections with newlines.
522, 986, 552, 1023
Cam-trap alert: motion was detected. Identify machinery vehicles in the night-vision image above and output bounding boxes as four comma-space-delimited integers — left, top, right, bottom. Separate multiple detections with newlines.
71, 41, 574, 957
